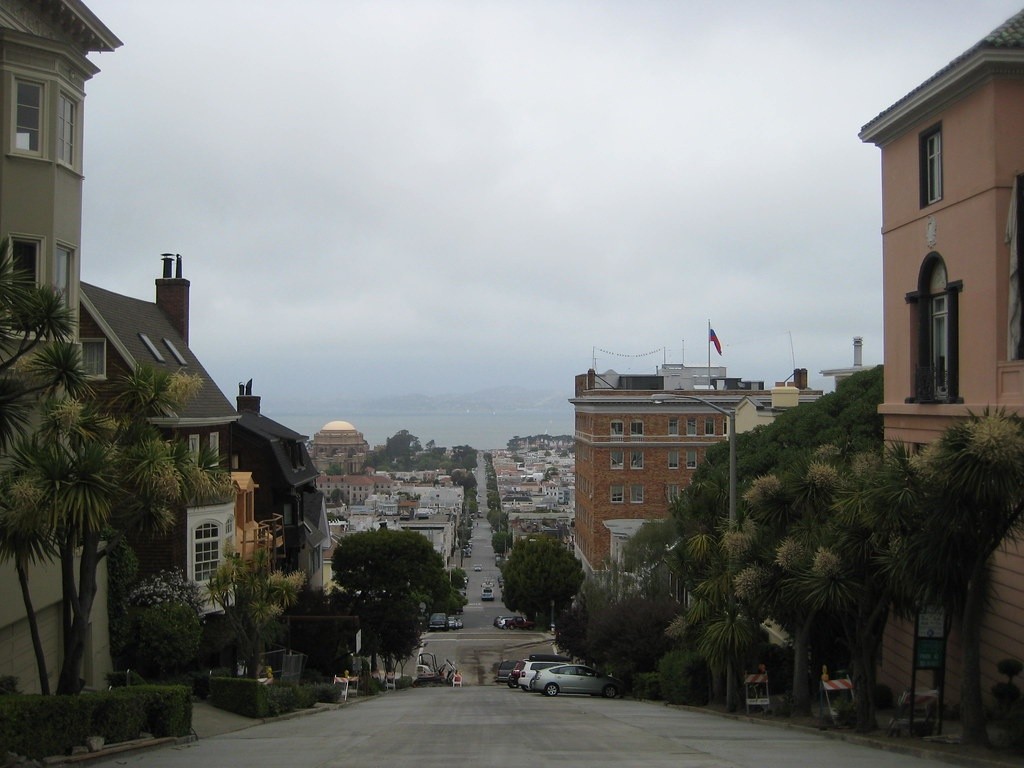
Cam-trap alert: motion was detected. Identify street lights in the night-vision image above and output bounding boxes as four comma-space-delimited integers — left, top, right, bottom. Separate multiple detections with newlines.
647, 392, 737, 714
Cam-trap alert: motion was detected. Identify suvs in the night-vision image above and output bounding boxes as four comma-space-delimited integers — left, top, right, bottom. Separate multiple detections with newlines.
428, 612, 451, 631
493, 658, 522, 689
493, 615, 527, 630
510, 652, 614, 692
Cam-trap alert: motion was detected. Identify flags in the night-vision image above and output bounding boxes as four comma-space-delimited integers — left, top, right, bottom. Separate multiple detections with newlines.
710, 329, 722, 356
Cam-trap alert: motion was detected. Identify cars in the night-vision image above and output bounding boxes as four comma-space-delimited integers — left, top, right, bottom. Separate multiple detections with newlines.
505, 616, 535, 630
465, 511, 485, 573
447, 576, 470, 630
529, 664, 626, 699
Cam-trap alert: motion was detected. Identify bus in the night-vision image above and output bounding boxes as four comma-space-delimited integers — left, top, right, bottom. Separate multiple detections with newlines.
480, 577, 495, 601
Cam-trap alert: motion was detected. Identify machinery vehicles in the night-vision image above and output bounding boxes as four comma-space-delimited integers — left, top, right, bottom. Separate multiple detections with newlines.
411, 651, 462, 688
